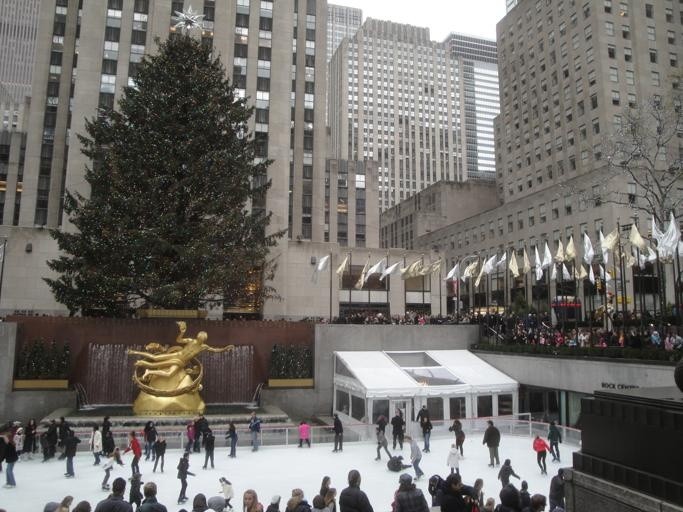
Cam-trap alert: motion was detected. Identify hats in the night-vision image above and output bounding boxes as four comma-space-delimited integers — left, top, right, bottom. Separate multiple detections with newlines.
398, 474, 411, 486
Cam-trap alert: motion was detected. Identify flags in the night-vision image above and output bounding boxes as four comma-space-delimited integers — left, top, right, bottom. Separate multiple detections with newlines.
316, 210, 682, 288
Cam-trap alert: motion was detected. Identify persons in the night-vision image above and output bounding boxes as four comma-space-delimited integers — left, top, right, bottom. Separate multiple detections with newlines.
17, 338, 71, 379
476, 302, 682, 393
1, 416, 80, 488
88, 413, 261, 503
125, 325, 233, 380
45, 467, 565, 511
298, 421, 310, 448
342, 405, 366, 421
374, 404, 562, 489
330, 413, 344, 452
320, 312, 482, 325
270, 344, 312, 378
